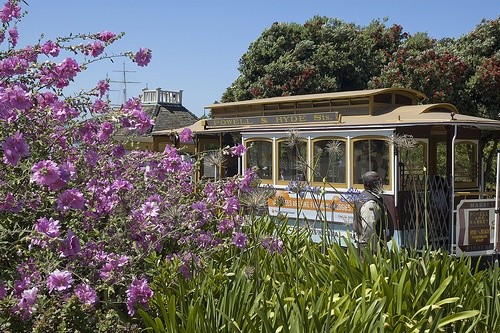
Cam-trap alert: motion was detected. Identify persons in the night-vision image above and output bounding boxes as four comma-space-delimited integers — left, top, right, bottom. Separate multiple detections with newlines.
353, 171, 395, 257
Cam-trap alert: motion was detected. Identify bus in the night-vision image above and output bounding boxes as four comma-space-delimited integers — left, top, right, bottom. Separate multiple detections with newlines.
152, 86, 500, 265
